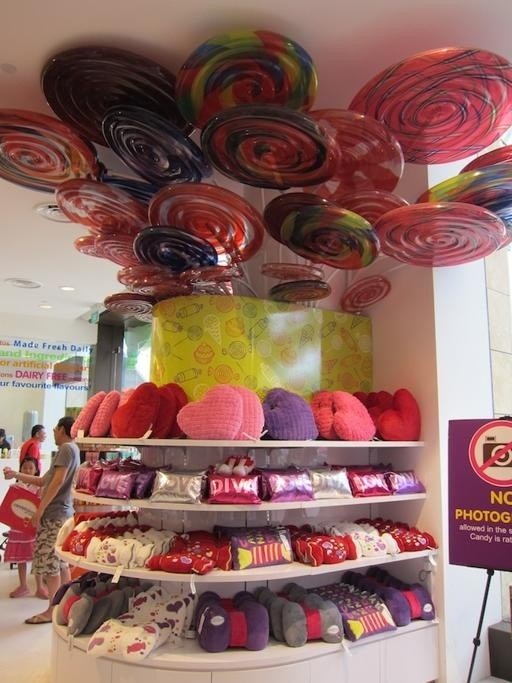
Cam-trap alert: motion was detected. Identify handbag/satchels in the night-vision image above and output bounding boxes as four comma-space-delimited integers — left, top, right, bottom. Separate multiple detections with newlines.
1, 485, 41, 537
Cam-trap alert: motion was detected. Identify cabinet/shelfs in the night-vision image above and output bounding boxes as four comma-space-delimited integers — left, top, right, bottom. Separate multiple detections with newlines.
52, 437, 439, 683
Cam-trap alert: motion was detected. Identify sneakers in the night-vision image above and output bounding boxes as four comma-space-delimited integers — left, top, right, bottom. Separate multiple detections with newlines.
10, 587, 30, 597
36, 589, 49, 599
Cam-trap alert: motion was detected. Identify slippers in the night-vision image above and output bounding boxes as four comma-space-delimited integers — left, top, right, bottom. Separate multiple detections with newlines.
25, 614, 52, 624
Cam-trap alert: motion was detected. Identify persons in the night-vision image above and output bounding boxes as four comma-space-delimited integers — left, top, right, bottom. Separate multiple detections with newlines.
0, 428, 11, 450
4, 415, 81, 624
17, 424, 47, 483
5, 455, 54, 600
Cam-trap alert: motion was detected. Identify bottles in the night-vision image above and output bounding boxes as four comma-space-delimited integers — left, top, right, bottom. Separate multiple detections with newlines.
3, 449, 9, 458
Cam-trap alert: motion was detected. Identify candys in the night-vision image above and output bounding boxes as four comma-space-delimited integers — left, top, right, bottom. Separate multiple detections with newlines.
0, 28, 512, 319
